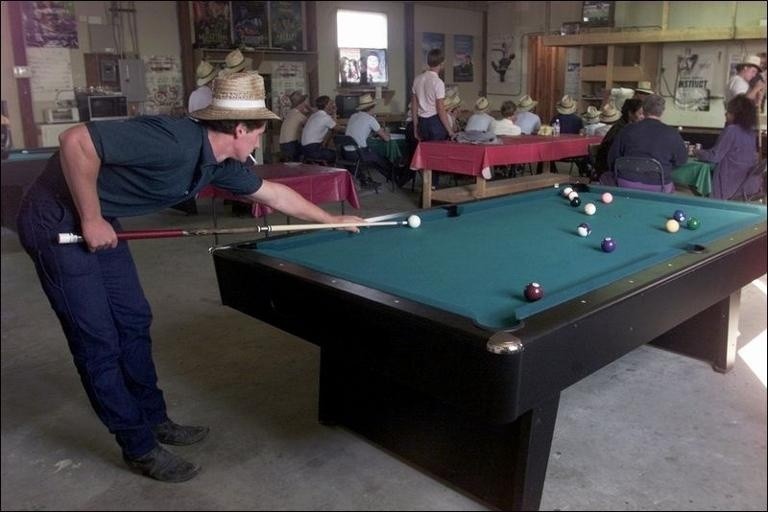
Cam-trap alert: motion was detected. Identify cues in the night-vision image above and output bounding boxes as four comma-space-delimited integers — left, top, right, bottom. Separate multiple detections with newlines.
758, 99, 762, 160
56, 221, 408, 243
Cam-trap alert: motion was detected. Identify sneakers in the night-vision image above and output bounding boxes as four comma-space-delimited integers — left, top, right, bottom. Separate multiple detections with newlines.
396, 173, 414, 187
123, 445, 201, 482
361, 178, 381, 189
151, 417, 212, 445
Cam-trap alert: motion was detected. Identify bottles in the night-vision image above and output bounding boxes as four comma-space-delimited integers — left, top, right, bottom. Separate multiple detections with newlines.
552, 119, 561, 137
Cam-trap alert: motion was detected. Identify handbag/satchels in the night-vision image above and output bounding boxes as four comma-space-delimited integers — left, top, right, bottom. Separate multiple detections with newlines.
452, 131, 498, 144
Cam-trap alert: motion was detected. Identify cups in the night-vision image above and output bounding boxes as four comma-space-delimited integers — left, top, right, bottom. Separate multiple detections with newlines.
579, 127, 593, 140
685, 141, 701, 157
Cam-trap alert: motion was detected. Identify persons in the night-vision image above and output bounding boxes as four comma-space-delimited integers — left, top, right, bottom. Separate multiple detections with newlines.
18, 73, 369, 483
692, 54, 766, 202
444, 87, 623, 176
412, 48, 458, 192
300, 95, 382, 189
188, 64, 216, 115
594, 81, 689, 194
279, 90, 314, 162
342, 94, 394, 181
339, 51, 384, 84
216, 49, 258, 216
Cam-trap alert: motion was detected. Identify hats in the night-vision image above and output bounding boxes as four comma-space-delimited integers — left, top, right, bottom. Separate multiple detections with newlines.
191, 73, 282, 121
442, 86, 463, 110
735, 55, 763, 72
196, 61, 217, 86
635, 81, 655, 94
472, 96, 491, 113
289, 91, 308, 109
555, 94, 621, 124
225, 49, 246, 73
516, 95, 538, 112
355, 93, 377, 110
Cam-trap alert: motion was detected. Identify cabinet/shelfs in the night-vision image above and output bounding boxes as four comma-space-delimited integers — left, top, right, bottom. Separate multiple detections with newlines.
563, 44, 662, 113
84, 52, 122, 93
35, 123, 84, 148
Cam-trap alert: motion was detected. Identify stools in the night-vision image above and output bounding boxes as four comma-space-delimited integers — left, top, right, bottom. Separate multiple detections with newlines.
276, 153, 299, 162
303, 159, 328, 166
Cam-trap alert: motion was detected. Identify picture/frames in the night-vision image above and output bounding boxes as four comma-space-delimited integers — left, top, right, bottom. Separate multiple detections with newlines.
581, 1, 615, 26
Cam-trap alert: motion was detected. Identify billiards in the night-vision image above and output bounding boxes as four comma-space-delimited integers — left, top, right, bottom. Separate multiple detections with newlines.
602, 193, 613, 203
601, 237, 616, 252
524, 282, 543, 301
408, 215, 421, 228
564, 188, 581, 207
577, 224, 592, 237
666, 210, 700, 232
584, 203, 596, 215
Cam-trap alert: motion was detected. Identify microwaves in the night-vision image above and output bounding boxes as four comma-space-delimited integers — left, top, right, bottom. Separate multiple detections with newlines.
77, 94, 130, 123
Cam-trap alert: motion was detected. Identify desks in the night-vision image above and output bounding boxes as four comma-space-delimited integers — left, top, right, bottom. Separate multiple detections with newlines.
322, 114, 408, 148
195, 162, 361, 245
213, 183, 767, 511
409, 134, 605, 210
367, 133, 406, 193
672, 157, 716, 196
0, 147, 60, 226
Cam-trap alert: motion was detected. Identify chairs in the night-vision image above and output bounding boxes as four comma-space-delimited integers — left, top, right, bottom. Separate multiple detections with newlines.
332, 135, 378, 195
614, 156, 664, 192
728, 158, 767, 204
588, 143, 601, 185
